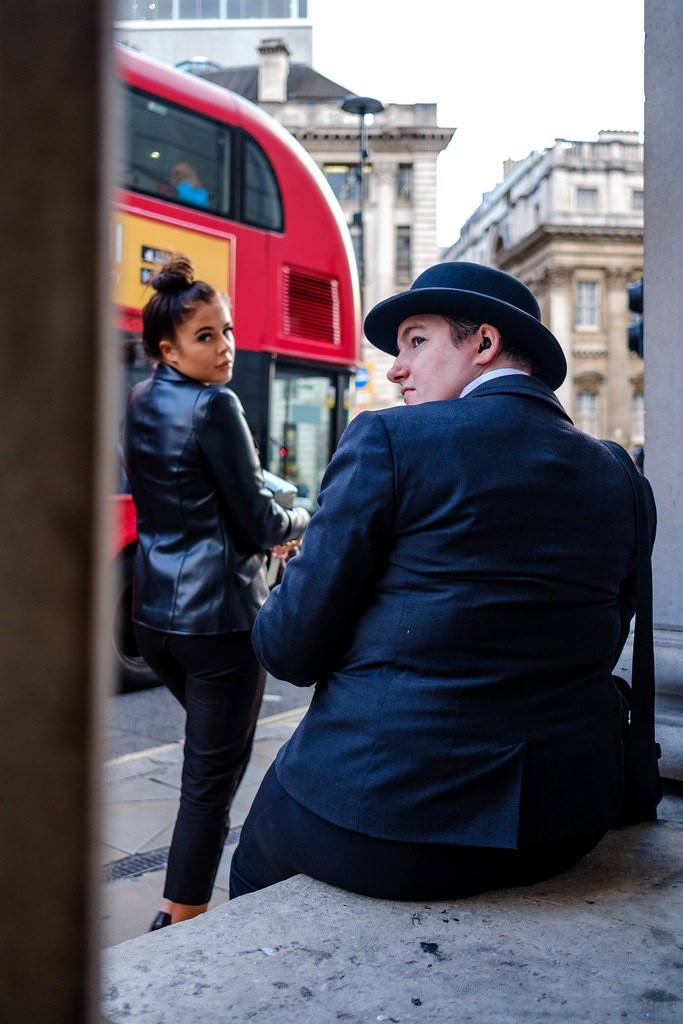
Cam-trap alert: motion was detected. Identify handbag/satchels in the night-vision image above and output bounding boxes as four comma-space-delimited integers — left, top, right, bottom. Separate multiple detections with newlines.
612, 675, 663, 827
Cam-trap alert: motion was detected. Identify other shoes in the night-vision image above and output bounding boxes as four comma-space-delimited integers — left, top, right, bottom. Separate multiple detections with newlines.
148, 911, 172, 933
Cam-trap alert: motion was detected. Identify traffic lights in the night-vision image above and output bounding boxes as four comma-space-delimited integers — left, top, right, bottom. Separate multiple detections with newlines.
623, 277, 647, 361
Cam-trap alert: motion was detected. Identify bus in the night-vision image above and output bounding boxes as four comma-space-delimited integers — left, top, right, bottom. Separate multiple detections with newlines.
109, 37, 364, 698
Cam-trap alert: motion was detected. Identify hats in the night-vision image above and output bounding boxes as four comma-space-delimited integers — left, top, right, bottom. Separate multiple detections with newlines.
364, 261, 567, 393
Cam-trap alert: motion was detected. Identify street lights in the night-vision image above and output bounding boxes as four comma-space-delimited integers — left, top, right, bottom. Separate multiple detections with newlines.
337, 94, 385, 326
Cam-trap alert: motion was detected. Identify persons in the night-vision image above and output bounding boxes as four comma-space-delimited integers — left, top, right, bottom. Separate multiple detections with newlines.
121, 257, 312, 932
168, 162, 208, 203
227, 261, 661, 897
